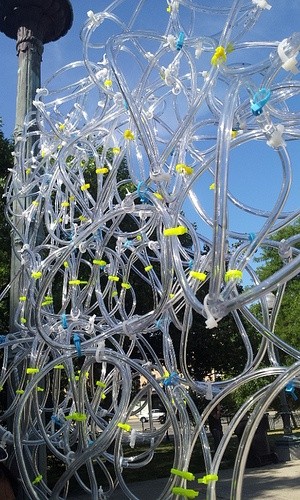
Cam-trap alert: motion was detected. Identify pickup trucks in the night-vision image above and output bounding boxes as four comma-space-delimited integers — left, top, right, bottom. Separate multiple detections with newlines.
140, 408, 168, 423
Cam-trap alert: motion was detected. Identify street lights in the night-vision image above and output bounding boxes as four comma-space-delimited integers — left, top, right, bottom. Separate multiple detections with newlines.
265, 292, 300, 444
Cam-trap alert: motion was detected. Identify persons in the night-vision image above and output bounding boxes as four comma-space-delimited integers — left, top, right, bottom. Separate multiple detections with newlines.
203, 397, 225, 462
